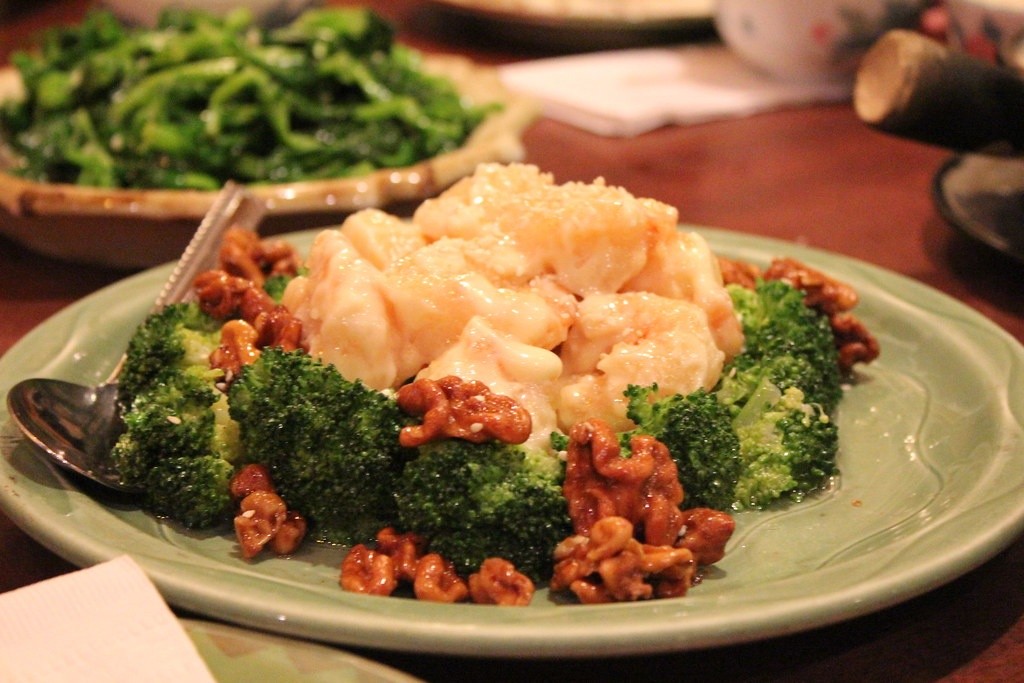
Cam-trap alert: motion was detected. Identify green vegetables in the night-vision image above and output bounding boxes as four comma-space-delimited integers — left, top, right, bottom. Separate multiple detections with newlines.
1, 5, 505, 193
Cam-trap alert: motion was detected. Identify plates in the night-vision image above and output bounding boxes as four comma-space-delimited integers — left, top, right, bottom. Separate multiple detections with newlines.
443, 0, 717, 30
0, 224, 1024, 658
175, 609, 428, 682
0, 48, 537, 267
934, 152, 1024, 260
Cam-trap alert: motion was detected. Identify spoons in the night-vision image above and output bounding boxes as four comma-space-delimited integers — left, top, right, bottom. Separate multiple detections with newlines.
7, 177, 266, 491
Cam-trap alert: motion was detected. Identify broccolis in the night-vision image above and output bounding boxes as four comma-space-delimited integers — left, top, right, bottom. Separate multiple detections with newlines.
111, 267, 846, 582
